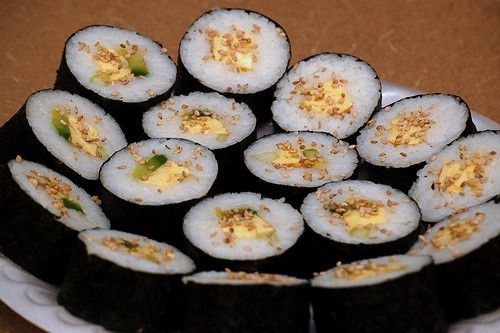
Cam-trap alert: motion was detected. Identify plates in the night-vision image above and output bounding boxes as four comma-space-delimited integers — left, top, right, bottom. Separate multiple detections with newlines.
0, 81, 500, 333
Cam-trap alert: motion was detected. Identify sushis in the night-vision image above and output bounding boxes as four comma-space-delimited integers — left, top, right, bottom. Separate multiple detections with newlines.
0, 6, 500, 333
